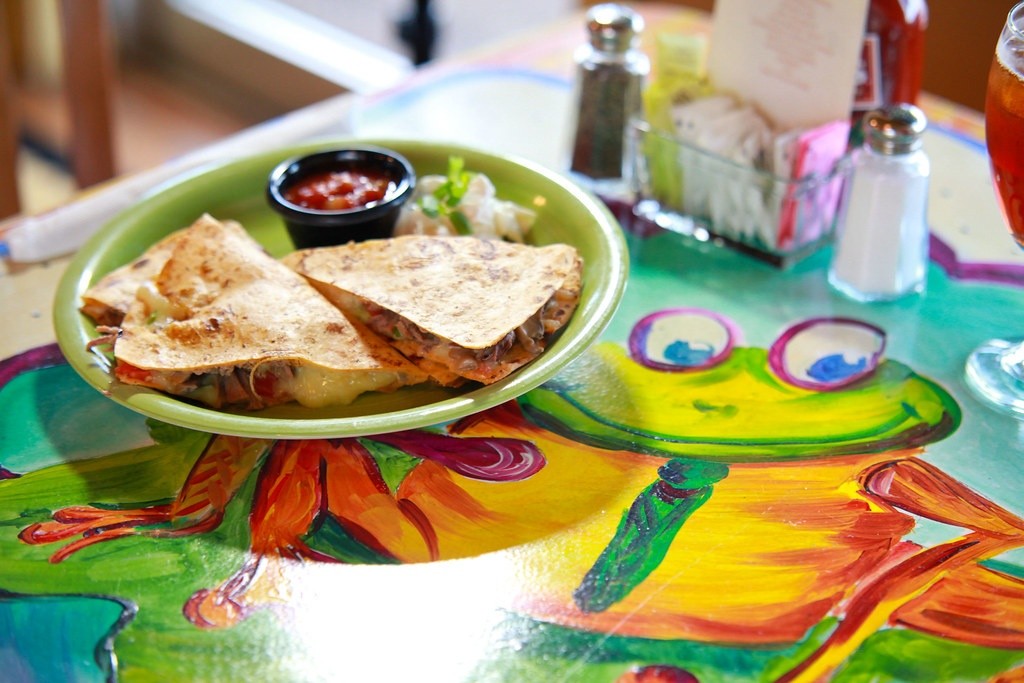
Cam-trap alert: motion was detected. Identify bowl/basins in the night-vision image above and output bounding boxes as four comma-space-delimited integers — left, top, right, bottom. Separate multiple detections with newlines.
264, 144, 417, 253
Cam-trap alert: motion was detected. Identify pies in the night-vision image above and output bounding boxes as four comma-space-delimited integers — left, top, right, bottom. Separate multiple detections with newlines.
78, 211, 583, 405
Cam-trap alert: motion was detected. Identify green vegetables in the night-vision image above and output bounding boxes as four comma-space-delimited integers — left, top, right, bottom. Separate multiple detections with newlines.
416, 156, 476, 235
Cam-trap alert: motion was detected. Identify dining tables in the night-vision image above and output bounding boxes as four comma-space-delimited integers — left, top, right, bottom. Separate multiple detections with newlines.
0, 0, 1024, 683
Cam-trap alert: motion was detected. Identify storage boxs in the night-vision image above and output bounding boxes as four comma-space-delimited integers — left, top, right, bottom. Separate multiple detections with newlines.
622, 112, 844, 268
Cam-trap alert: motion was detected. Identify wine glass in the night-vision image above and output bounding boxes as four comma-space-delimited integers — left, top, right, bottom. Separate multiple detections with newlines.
964, 1, 1024, 426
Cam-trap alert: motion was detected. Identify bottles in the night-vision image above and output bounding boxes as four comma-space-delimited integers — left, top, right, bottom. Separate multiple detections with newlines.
827, 102, 929, 302
852, 0, 931, 130
566, 4, 649, 201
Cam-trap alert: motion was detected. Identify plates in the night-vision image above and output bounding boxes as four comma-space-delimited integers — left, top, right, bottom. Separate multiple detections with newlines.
54, 135, 631, 439
